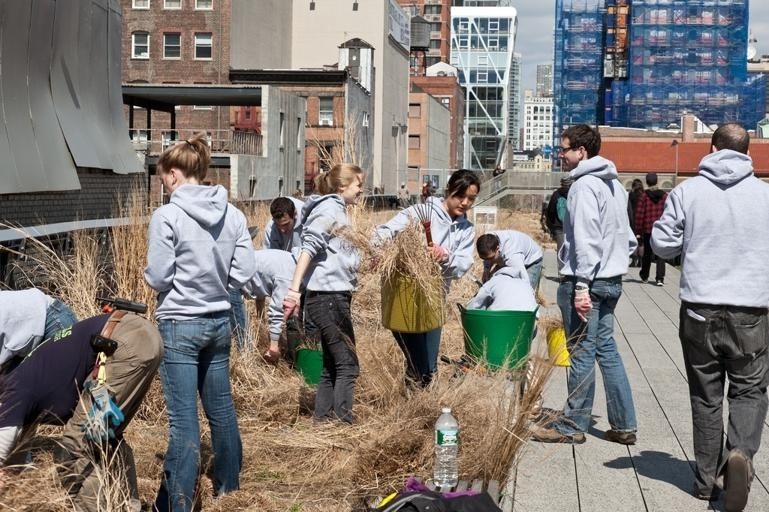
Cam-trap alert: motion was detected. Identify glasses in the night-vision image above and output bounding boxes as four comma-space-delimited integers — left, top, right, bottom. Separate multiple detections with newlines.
559, 146, 580, 155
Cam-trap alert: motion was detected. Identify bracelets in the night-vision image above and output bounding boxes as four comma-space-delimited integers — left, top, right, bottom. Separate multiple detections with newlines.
575, 286, 588, 290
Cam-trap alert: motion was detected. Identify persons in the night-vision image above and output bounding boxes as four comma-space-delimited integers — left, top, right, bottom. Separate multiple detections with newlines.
144, 131, 258, 510
531, 121, 638, 445
370, 169, 480, 391
397, 181, 410, 208
650, 122, 769, 512
419, 180, 448, 201
262, 197, 318, 362
0, 288, 78, 369
635, 171, 668, 286
466, 264, 540, 339
547, 176, 572, 256
280, 162, 367, 426
476, 229, 544, 289
493, 165, 506, 188
627, 178, 644, 267
1, 309, 166, 510
229, 245, 303, 358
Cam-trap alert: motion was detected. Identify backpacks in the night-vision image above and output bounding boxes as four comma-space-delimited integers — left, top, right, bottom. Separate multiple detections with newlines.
556, 195, 567, 223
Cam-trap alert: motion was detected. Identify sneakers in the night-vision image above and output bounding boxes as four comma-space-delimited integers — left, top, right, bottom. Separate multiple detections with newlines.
698, 476, 723, 500
723, 451, 750, 512
532, 428, 586, 443
639, 270, 649, 283
655, 276, 664, 286
604, 430, 637, 445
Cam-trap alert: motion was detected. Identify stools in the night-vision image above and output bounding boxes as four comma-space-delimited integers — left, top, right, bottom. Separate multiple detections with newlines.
409, 475, 499, 508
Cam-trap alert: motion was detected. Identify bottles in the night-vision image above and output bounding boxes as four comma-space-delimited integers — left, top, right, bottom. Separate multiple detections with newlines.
432, 408, 460, 491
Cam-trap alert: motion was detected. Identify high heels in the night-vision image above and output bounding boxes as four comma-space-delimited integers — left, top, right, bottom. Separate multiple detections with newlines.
629, 259, 637, 267
637, 259, 642, 267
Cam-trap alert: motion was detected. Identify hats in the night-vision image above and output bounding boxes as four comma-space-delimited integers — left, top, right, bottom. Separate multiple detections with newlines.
560, 177, 572, 186
646, 173, 658, 186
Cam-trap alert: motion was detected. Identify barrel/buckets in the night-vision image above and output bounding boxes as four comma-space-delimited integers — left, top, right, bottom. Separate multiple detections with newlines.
545, 327, 572, 366
380, 265, 446, 334
293, 346, 325, 385
456, 302, 540, 372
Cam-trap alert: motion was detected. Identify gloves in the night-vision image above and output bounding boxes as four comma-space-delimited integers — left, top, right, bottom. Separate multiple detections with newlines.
282, 287, 302, 320
574, 288, 593, 324
427, 242, 450, 266
81, 383, 125, 448
